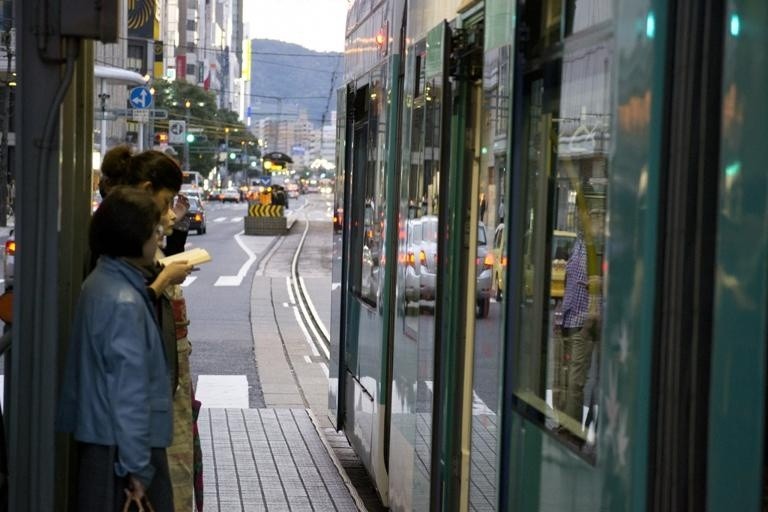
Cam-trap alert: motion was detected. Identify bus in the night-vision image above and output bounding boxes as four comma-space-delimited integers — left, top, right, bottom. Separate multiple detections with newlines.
327, 0, 767, 512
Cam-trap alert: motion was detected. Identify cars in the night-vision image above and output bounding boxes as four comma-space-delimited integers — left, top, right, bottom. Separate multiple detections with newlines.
171, 172, 242, 233
3, 229, 16, 292
487, 219, 579, 312
362, 214, 495, 321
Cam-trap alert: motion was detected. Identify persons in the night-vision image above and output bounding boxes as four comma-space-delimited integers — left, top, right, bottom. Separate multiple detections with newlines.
51, 185, 176, 512
157, 193, 204, 512
547, 192, 605, 456
98, 145, 193, 399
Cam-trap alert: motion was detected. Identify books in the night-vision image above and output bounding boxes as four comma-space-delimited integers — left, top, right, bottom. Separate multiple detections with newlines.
157, 248, 211, 269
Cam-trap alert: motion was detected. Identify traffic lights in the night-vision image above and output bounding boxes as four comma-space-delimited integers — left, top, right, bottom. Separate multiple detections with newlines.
264, 159, 272, 171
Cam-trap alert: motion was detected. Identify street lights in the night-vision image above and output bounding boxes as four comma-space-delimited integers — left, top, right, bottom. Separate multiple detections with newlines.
184, 99, 191, 172
225, 128, 229, 175
